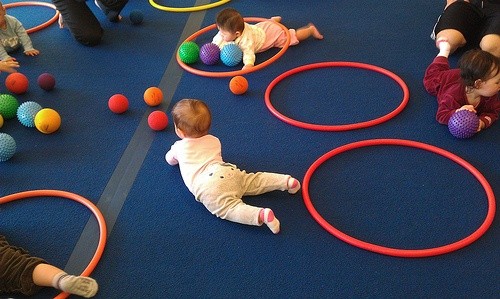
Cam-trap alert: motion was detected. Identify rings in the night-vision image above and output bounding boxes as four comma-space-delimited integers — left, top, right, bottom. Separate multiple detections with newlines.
5, 61, 6, 64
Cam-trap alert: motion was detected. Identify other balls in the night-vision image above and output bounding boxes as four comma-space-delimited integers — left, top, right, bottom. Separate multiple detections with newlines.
108, 93, 129, 114
35, 108, 62, 135
148, 110, 168, 131
143, 86, 164, 106
228, 76, 249, 97
37, 72, 55, 90
130, 10, 144, 24
5, 72, 29, 94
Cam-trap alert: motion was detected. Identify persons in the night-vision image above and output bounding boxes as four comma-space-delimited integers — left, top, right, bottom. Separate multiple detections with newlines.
165, 99, 301, 234
424, 37, 500, 132
0, 58, 19, 73
0, 2, 40, 63
430, 0, 500, 59
51, 0, 130, 47
211, 8, 323, 71
0, 233, 98, 297
